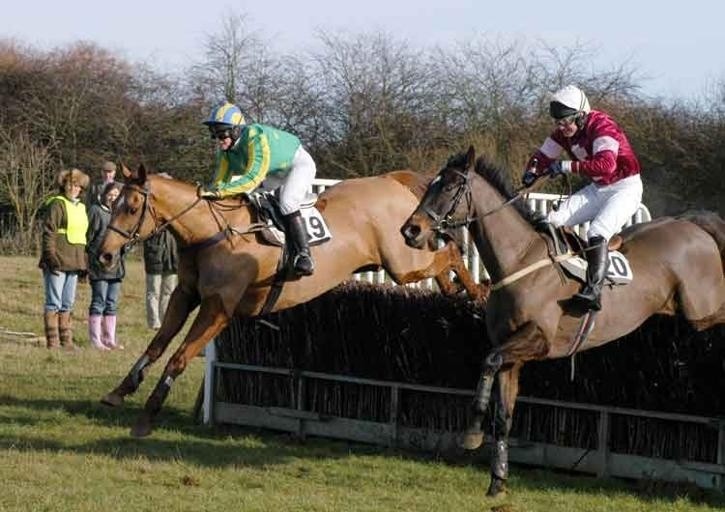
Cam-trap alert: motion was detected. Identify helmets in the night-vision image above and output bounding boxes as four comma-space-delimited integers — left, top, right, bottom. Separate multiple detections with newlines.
202, 103, 247, 126
550, 85, 591, 119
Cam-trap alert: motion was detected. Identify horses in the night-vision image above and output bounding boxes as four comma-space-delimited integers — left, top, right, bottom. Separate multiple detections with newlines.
398, 145, 724, 501
98, 157, 494, 437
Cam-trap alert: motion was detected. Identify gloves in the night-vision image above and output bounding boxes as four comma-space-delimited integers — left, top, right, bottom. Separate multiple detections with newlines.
47, 254, 61, 267
197, 184, 220, 200
522, 171, 537, 188
543, 160, 562, 179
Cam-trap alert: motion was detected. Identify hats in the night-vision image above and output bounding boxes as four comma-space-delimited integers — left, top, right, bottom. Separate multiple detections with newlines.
58, 168, 90, 191
102, 161, 116, 171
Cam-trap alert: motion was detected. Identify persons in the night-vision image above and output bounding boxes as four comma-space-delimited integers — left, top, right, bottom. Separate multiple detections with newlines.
87, 183, 125, 352
39, 167, 90, 351
145, 172, 178, 328
200, 100, 321, 279
524, 83, 645, 310
93, 162, 122, 192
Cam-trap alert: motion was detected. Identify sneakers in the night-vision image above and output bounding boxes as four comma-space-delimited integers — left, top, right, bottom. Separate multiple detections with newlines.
147, 318, 161, 329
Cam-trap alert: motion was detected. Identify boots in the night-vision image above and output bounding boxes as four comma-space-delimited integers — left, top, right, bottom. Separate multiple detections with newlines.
101, 315, 124, 350
44, 311, 62, 350
59, 311, 74, 352
290, 216, 314, 276
88, 314, 111, 351
572, 236, 609, 310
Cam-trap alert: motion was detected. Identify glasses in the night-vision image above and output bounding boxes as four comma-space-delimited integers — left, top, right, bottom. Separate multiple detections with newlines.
550, 115, 575, 127
210, 134, 230, 140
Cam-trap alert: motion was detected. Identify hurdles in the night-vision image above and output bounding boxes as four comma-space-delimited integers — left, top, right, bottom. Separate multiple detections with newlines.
201, 281, 725, 494
